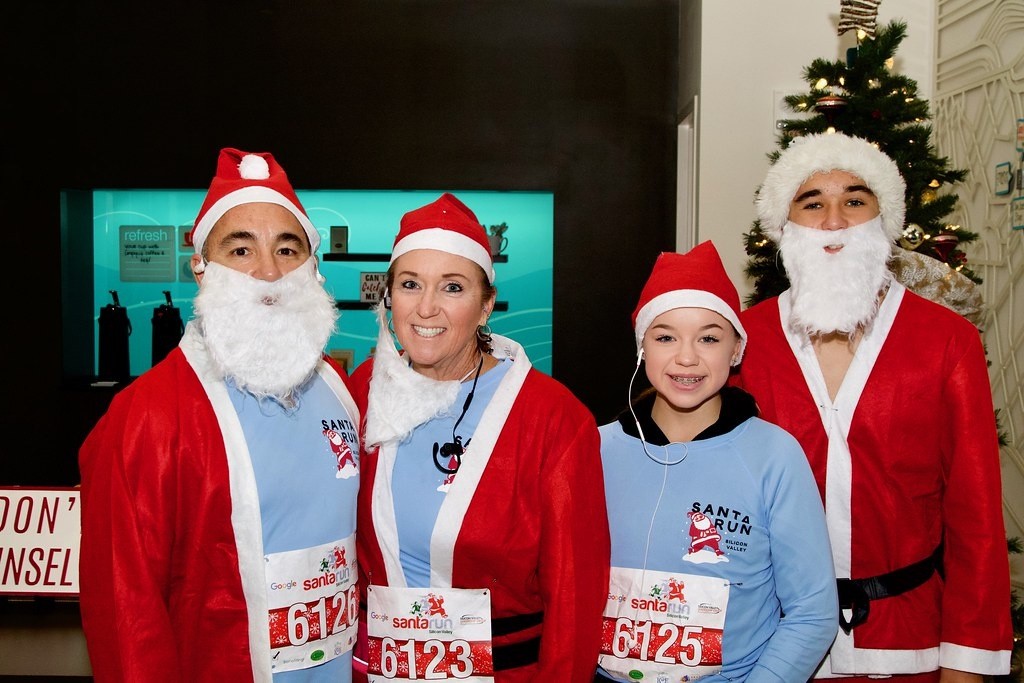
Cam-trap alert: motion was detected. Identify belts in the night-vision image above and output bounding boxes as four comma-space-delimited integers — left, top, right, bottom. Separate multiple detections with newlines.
835, 552, 935, 631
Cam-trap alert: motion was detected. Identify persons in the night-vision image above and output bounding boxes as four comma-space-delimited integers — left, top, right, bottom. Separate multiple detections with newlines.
729, 128, 1014, 683
347, 193, 612, 683
78, 146, 361, 683
597, 241, 840, 683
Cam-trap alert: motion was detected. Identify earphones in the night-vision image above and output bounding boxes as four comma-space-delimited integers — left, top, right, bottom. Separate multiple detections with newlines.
637, 348, 646, 366
441, 442, 463, 457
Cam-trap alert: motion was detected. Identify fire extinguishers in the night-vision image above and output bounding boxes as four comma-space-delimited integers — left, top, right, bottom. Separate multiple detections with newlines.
97, 291, 133, 381
151, 289, 186, 369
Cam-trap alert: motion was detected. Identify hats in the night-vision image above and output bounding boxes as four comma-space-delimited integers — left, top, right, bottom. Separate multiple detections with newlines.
192, 148, 320, 256
756, 129, 906, 244
631, 240, 748, 366
388, 194, 495, 287
